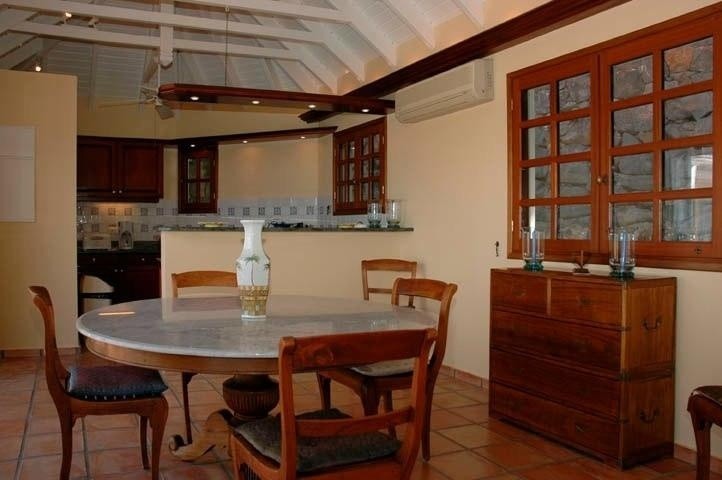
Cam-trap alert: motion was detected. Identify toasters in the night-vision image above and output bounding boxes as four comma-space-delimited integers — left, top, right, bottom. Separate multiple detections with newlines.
82, 231, 112, 251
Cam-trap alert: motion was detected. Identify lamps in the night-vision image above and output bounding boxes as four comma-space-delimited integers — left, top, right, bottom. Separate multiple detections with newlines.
88, 17, 99, 28
62, 12, 72, 24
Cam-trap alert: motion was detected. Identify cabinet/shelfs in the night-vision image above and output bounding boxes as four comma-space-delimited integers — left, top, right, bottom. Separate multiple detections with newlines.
489, 269, 678, 471
77, 135, 164, 204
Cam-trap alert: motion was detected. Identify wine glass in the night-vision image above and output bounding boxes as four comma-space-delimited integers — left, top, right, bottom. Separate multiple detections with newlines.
572, 250, 592, 273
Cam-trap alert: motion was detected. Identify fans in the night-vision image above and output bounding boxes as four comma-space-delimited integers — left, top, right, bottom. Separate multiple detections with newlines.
99, 64, 176, 121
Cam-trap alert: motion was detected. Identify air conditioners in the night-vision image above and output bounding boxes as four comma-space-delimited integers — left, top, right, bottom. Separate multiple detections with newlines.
393, 58, 499, 123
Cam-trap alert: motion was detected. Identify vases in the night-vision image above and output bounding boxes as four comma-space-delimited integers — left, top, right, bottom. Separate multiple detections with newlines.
235, 219, 272, 319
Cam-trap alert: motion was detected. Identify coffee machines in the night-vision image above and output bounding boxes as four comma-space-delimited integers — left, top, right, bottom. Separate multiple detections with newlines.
119, 221, 134, 250
